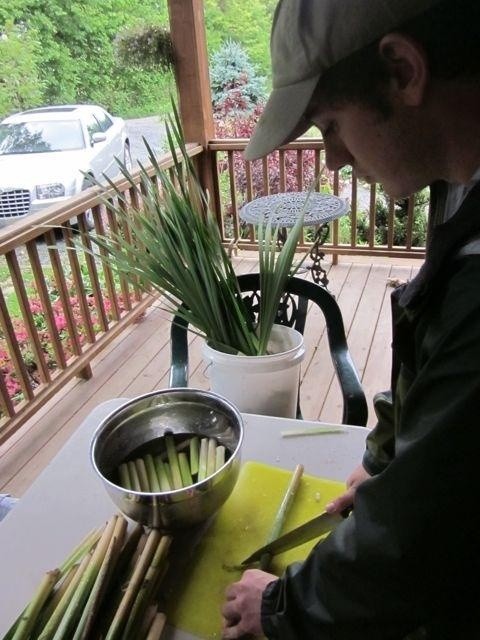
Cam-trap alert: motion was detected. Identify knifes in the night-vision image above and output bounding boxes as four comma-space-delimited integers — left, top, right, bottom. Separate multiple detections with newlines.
240, 499, 353, 566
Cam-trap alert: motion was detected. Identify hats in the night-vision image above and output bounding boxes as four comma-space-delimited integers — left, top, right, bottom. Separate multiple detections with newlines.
236, 0, 438, 166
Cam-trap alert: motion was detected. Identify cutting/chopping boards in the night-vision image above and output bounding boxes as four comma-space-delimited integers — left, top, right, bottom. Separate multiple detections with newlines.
155, 459, 350, 640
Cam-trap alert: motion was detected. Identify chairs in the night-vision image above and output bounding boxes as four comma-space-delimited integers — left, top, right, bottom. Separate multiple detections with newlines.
169, 274, 370, 426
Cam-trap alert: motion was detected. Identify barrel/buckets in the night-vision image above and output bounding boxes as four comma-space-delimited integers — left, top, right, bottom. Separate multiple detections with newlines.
201, 324, 305, 420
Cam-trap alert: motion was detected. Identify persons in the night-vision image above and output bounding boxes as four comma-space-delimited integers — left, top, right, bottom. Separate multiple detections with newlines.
220, 2, 479, 640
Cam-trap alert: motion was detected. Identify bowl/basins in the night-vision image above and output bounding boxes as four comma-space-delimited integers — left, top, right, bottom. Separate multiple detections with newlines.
89, 386, 246, 534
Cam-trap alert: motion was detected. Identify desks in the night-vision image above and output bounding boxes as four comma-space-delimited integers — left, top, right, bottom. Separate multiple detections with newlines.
1, 396, 373, 639
238, 191, 350, 302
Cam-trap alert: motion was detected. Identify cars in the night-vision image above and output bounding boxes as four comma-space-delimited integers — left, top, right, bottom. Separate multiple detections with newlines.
0, 104, 131, 232
